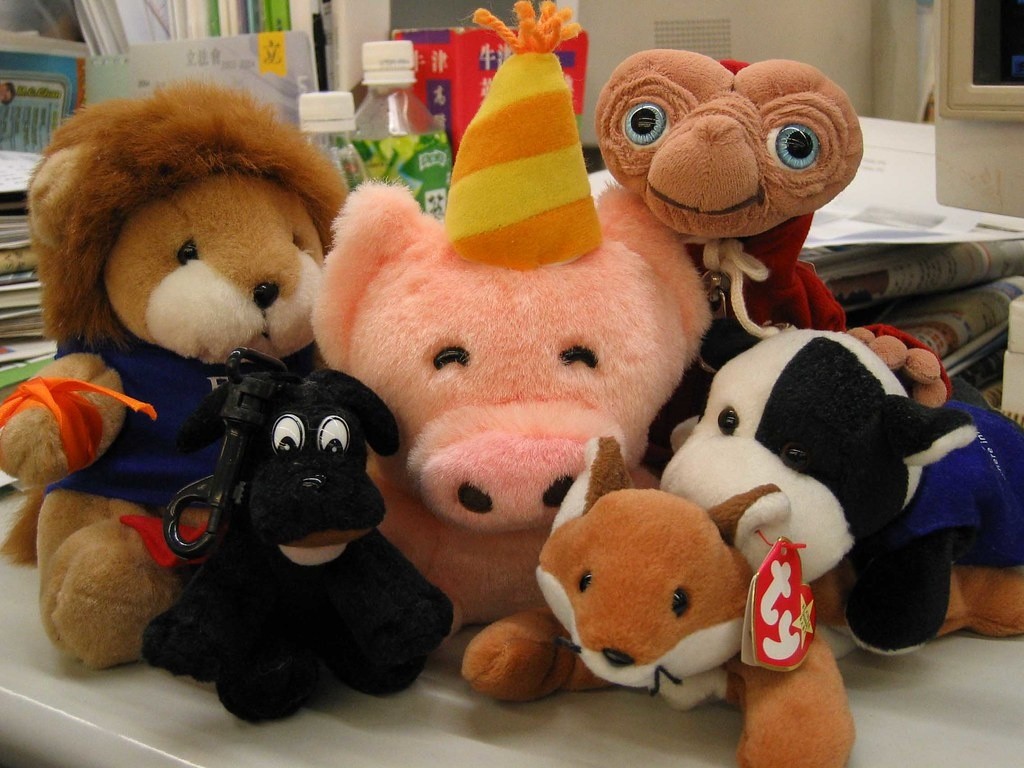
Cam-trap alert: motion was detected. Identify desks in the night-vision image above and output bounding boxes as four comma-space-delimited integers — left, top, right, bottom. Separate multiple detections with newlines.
2, 484, 1024, 768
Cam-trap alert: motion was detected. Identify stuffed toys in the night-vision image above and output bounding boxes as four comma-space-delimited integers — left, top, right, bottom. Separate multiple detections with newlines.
311, 0, 709, 628
599, 44, 952, 472
1, 73, 348, 674
661, 329, 1024, 656
141, 345, 456, 728
463, 435, 1024, 757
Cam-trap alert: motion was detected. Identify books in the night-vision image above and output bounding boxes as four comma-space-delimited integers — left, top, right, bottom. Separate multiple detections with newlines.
0, 0, 292, 378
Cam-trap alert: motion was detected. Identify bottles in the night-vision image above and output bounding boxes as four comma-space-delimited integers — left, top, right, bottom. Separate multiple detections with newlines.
298, 89, 366, 208
351, 38, 454, 223
